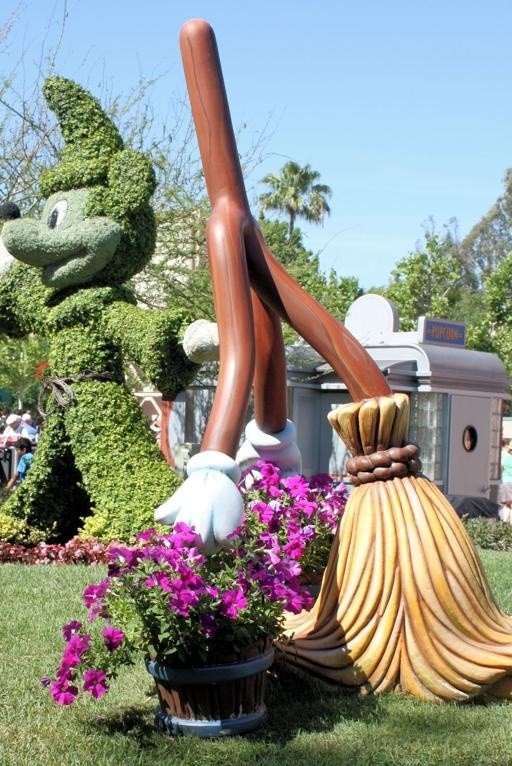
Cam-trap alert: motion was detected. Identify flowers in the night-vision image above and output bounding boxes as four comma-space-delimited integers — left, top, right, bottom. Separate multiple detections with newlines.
38, 519, 289, 704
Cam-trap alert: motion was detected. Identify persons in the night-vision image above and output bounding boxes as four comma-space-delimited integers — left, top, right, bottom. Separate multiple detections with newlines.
500, 437, 512, 482
0, 409, 42, 491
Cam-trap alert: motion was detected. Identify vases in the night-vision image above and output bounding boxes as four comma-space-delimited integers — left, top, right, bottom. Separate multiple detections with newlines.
142, 635, 277, 738
